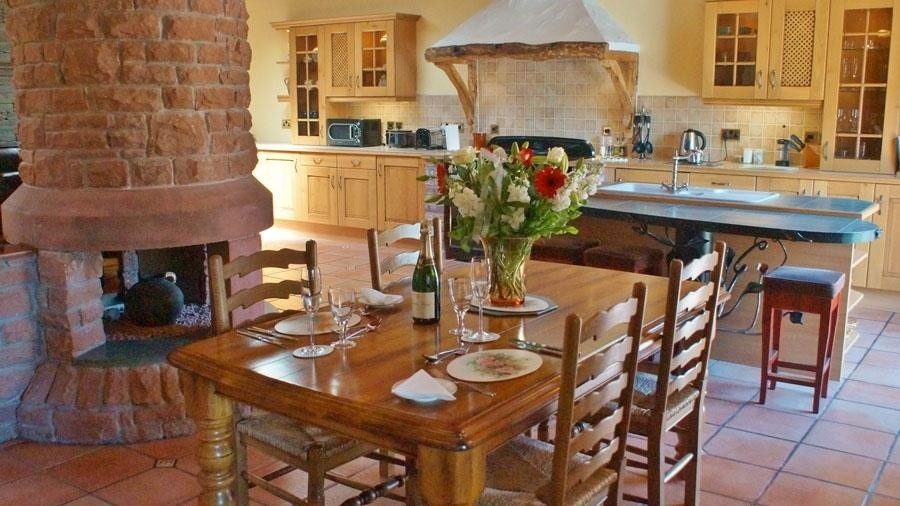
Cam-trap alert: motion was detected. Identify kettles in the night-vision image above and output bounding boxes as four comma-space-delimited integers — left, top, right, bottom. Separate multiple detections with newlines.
679, 128, 705, 161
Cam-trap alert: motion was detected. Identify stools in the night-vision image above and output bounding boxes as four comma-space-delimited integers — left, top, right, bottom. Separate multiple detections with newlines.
534, 231, 603, 270
579, 242, 664, 278
756, 264, 847, 415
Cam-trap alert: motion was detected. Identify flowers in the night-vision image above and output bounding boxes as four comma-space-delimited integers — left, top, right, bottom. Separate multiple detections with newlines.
411, 136, 608, 300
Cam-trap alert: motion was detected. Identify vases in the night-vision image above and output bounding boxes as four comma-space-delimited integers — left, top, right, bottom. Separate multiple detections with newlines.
478, 230, 540, 309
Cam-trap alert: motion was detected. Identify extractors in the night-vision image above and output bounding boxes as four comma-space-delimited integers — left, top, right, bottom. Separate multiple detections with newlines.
424, 0, 639, 64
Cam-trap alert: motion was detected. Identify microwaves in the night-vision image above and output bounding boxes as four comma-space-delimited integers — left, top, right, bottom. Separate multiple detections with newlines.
325, 117, 382, 148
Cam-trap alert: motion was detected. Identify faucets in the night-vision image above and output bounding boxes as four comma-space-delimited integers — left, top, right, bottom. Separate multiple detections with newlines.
672, 147, 705, 195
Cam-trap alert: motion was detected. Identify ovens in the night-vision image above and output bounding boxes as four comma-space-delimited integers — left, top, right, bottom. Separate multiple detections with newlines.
442, 163, 495, 260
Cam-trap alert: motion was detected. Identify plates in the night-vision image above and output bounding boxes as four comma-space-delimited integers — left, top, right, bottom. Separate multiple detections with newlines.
391, 378, 458, 405
361, 295, 406, 309
719, 26, 753, 35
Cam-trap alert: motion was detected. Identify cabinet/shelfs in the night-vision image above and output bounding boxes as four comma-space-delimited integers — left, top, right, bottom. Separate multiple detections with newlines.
817, 0, 900, 179
700, 0, 831, 106
322, 12, 421, 102
591, 162, 690, 190
689, 167, 756, 194
269, 17, 353, 146
866, 176, 900, 295
376, 148, 430, 237
754, 172, 875, 290
843, 245, 868, 359
247, 145, 297, 230
298, 144, 377, 233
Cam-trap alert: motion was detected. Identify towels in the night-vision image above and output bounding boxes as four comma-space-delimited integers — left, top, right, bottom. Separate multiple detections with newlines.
356, 286, 406, 309
389, 364, 458, 407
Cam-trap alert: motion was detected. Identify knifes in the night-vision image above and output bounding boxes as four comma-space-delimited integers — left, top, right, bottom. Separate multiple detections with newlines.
508, 336, 581, 362
228, 324, 299, 351
787, 135, 804, 152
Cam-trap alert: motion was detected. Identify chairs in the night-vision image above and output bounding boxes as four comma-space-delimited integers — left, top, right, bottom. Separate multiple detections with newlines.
364, 217, 445, 498
203, 241, 397, 506
398, 281, 650, 506
536, 233, 729, 506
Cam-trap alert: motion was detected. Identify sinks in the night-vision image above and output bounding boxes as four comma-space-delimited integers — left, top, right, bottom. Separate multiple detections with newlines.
691, 184, 780, 203
596, 181, 670, 197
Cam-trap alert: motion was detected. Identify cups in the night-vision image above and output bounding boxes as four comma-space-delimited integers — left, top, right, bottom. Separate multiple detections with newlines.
844, 39, 875, 49
473, 132, 486, 150
719, 51, 731, 64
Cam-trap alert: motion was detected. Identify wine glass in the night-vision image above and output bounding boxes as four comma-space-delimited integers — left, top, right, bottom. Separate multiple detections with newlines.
445, 278, 473, 336
462, 254, 501, 344
296, 268, 329, 358
835, 57, 861, 132
327, 285, 359, 350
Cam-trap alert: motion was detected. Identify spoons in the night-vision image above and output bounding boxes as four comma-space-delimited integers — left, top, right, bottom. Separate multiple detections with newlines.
348, 316, 384, 342
422, 343, 473, 362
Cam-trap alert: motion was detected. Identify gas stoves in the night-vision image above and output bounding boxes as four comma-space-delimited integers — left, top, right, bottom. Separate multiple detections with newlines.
446, 151, 587, 174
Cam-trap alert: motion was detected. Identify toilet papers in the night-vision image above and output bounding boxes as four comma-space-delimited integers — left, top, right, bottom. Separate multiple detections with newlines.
444, 124, 461, 152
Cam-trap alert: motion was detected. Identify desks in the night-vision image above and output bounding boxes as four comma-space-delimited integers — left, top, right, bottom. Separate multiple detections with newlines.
570, 192, 881, 384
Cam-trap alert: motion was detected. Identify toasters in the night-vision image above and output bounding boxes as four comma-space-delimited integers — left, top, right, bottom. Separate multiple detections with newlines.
414, 126, 444, 150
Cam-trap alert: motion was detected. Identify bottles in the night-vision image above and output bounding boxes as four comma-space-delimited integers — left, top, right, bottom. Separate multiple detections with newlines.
411, 220, 442, 326
606, 135, 614, 158
599, 135, 606, 158
753, 148, 764, 164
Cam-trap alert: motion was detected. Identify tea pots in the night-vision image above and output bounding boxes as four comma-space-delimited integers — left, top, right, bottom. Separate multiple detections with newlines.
775, 138, 789, 166
